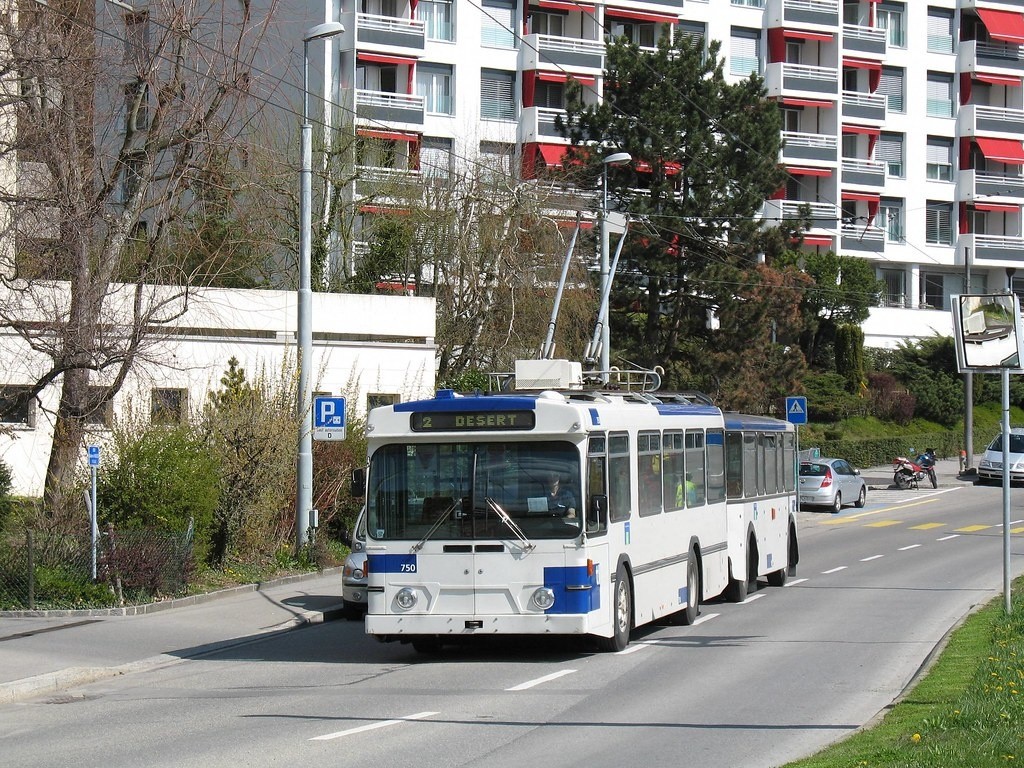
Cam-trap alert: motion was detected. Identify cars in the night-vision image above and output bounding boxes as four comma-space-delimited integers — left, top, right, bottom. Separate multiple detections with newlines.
342, 497, 448, 619
798, 458, 867, 512
978, 428, 1024, 488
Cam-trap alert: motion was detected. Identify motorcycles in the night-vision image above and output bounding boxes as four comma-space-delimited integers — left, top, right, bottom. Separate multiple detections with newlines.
893, 447, 939, 489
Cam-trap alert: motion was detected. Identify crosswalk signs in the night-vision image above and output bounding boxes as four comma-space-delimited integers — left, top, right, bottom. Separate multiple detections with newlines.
786, 397, 809, 426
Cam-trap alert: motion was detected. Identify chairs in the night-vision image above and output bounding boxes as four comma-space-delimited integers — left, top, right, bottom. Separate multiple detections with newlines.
421, 497, 452, 525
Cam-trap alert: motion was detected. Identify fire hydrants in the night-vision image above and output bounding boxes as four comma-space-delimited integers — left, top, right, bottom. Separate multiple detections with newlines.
959, 450, 967, 472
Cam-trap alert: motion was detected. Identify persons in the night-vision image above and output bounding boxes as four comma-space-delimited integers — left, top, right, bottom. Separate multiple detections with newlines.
676, 473, 697, 507
539, 471, 576, 518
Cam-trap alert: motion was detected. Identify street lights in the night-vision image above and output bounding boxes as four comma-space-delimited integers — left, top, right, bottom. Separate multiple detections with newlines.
602, 151, 632, 369
296, 21, 344, 560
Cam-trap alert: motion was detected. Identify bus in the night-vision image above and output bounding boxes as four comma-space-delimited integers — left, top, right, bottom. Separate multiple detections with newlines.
365, 360, 729, 653
723, 412, 799, 602
